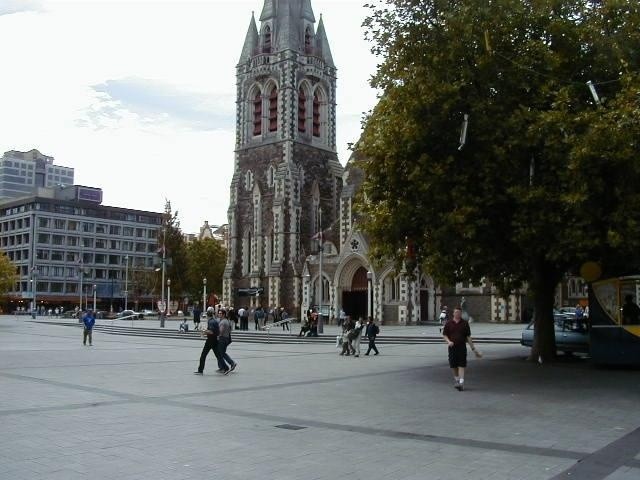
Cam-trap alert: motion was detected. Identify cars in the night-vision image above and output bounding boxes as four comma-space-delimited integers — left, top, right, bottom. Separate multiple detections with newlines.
116, 309, 156, 319
520, 314, 589, 355
559, 306, 588, 320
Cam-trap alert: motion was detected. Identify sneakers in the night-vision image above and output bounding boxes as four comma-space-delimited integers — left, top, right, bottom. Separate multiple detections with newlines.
340, 350, 378, 357
216, 363, 236, 375
194, 371, 203, 374
455, 383, 464, 391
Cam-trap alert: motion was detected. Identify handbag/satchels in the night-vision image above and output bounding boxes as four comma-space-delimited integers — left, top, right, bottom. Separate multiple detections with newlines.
348, 329, 360, 339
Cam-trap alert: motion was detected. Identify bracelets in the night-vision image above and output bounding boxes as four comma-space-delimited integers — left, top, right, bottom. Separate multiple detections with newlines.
472, 348, 476, 350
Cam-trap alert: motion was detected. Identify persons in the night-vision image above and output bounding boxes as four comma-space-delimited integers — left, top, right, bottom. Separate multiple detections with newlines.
443, 307, 482, 392
439, 306, 448, 332
55, 307, 59, 319
216, 310, 237, 374
74, 305, 80, 319
47, 307, 53, 319
556, 304, 590, 332
191, 303, 319, 337
622, 294, 640, 326
82, 310, 95, 346
329, 307, 380, 358
178, 317, 188, 334
194, 309, 230, 376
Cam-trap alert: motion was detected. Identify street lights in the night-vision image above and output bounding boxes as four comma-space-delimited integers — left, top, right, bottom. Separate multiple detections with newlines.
92, 283, 97, 311
78, 243, 85, 310
366, 270, 373, 323
167, 279, 172, 316
123, 254, 130, 309
201, 276, 208, 318
159, 227, 168, 328
316, 206, 326, 334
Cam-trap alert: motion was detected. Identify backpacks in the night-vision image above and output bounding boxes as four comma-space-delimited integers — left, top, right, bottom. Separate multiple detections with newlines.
372, 323, 379, 334
